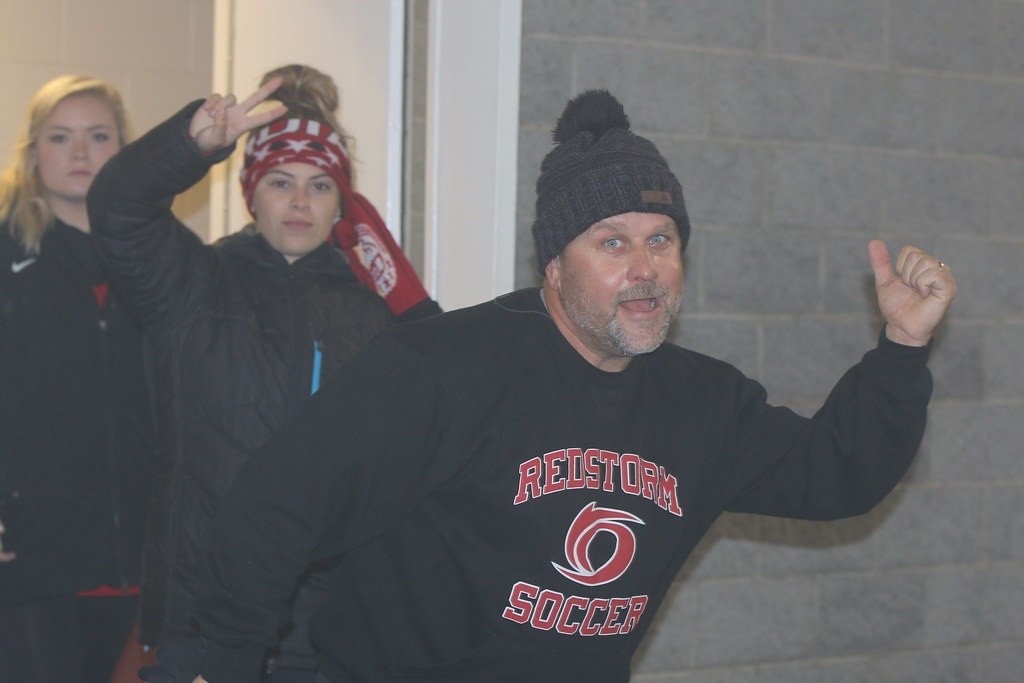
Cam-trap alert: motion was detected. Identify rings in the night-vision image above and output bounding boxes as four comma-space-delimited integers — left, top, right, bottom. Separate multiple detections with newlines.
936, 257, 945, 271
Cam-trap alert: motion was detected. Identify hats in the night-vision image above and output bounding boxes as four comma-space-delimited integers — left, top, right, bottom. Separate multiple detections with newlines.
532, 89, 690, 276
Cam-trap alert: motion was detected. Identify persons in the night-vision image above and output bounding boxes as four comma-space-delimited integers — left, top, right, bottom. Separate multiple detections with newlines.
80, 64, 443, 682
0, 74, 153, 683
157, 89, 958, 683
